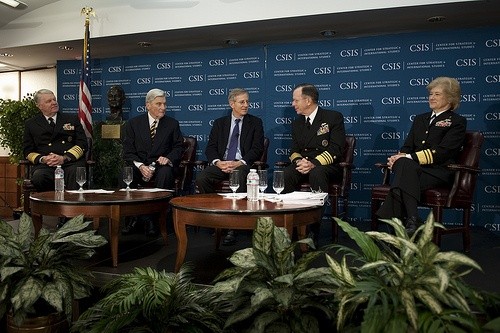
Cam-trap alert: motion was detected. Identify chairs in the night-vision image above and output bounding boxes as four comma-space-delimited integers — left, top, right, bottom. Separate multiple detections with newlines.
132, 137, 197, 198
371, 130, 484, 248
275, 136, 355, 244
194, 137, 268, 233
19, 137, 95, 216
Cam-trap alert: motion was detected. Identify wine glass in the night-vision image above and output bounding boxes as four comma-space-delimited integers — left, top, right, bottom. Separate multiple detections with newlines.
258, 170, 268, 199
76, 167, 86, 191
272, 171, 284, 201
123, 167, 133, 191
228, 170, 240, 197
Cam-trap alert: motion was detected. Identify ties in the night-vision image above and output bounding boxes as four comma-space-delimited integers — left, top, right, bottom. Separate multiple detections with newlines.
48, 118, 56, 129
306, 117, 311, 130
227, 119, 240, 161
150, 121, 157, 148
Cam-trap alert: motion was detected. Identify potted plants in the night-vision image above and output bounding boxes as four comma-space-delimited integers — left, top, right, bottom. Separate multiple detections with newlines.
0, 211, 108, 333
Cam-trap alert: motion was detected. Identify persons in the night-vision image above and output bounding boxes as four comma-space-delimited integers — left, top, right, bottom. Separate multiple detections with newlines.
196, 88, 265, 246
121, 88, 184, 238
270, 83, 347, 251
376, 76, 467, 257
23, 89, 90, 236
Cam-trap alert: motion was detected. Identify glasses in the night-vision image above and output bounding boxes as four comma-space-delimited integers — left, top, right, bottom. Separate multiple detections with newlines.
233, 100, 249, 105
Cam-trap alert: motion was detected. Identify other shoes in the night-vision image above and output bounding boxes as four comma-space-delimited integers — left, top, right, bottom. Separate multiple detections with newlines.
222, 234, 233, 245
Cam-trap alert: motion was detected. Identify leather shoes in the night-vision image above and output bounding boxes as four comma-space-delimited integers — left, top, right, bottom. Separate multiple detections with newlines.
405, 215, 417, 232
121, 219, 144, 235
55, 217, 68, 230
147, 220, 160, 238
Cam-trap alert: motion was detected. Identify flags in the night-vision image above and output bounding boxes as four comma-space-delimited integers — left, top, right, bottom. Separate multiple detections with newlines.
77, 20, 93, 162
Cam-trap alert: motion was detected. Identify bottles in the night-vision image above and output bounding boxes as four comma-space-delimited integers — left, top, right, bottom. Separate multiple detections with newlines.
55, 166, 64, 192
247, 169, 260, 201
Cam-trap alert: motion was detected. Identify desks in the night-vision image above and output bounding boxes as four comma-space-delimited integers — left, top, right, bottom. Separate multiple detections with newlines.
29, 191, 173, 267
169, 194, 324, 273
